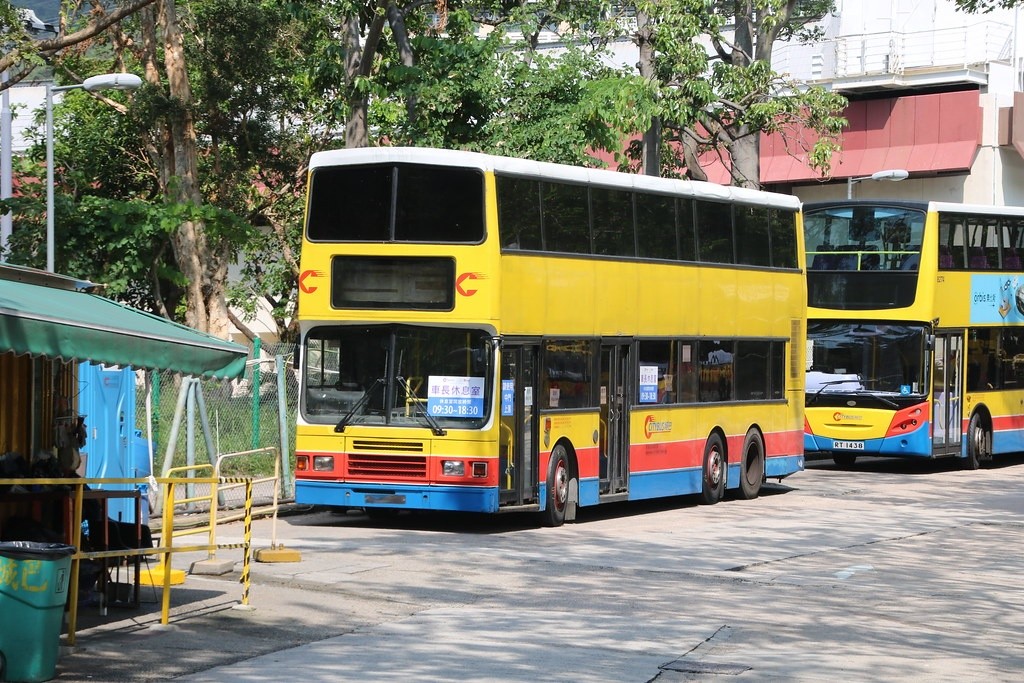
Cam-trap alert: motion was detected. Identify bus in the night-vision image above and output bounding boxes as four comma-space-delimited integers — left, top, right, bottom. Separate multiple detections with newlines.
288, 145, 810, 528
797, 198, 1024, 473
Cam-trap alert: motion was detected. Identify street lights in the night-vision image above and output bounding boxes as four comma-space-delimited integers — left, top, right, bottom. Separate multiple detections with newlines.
45, 72, 145, 275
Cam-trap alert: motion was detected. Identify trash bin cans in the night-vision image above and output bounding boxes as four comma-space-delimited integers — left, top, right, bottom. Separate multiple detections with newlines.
0, 541, 77, 683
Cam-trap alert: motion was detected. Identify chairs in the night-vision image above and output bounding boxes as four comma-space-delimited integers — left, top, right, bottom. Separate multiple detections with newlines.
811, 244, 1024, 272
81, 520, 155, 609
